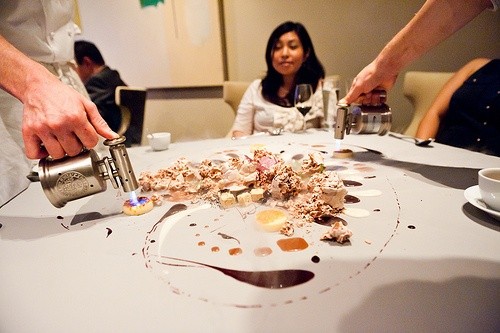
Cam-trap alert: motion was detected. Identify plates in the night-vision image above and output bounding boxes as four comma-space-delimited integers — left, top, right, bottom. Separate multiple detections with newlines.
463, 184, 500, 217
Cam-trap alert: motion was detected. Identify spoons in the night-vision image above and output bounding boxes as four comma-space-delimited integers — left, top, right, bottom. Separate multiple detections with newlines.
389, 133, 432, 146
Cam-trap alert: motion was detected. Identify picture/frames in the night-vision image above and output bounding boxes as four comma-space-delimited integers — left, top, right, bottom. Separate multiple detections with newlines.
75, 0, 229, 90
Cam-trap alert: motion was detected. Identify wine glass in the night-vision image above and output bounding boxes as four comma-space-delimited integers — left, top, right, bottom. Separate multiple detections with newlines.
294, 84, 314, 134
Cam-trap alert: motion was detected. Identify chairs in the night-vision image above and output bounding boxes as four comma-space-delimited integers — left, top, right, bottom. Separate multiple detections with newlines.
114, 85, 148, 149
224, 73, 340, 133
401, 71, 457, 139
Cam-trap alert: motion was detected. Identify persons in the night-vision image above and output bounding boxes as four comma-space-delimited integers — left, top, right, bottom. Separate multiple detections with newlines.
340, 0, 500, 157
0, 0, 120, 209
224, 21, 340, 140
73, 40, 129, 133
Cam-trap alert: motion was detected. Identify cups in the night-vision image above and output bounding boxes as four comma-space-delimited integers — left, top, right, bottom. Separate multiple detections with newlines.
147, 132, 171, 151
321, 80, 340, 132
478, 167, 500, 212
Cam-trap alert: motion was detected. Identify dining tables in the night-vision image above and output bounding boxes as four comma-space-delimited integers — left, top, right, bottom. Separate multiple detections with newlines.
0, 126, 499, 331
